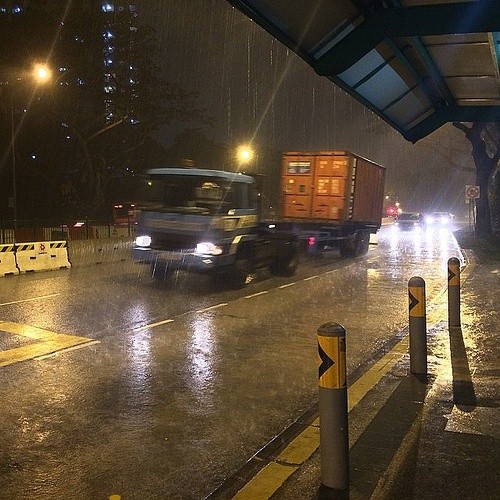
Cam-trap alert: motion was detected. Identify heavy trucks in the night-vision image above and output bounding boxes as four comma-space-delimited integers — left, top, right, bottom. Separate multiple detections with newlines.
128, 148, 387, 290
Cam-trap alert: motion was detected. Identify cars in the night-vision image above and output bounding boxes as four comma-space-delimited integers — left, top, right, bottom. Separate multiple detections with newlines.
394, 212, 427, 234
427, 211, 456, 228
